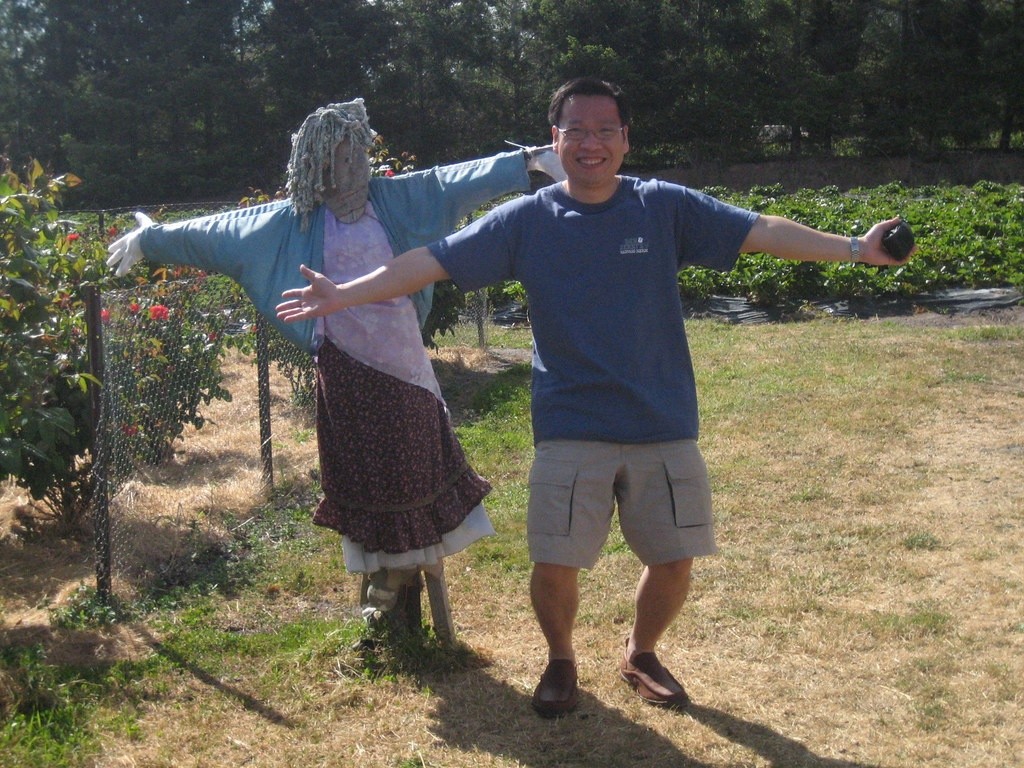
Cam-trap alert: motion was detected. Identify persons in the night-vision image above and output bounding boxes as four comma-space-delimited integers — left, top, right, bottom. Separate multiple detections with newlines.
102, 97, 566, 651
277, 76, 918, 720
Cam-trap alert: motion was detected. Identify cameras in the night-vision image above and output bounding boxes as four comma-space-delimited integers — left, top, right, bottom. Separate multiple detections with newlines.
883, 222, 914, 260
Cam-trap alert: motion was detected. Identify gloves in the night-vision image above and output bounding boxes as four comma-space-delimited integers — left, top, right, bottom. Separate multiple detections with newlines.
106, 212, 160, 278
527, 146, 568, 183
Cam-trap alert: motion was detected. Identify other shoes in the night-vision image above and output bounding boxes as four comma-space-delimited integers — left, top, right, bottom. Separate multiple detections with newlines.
618, 637, 690, 710
532, 658, 581, 717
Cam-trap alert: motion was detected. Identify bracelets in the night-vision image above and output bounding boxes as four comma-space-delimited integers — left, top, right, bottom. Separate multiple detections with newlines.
851, 236, 859, 263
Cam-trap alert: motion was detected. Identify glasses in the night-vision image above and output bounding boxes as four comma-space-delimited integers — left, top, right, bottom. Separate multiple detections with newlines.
558, 127, 623, 141
